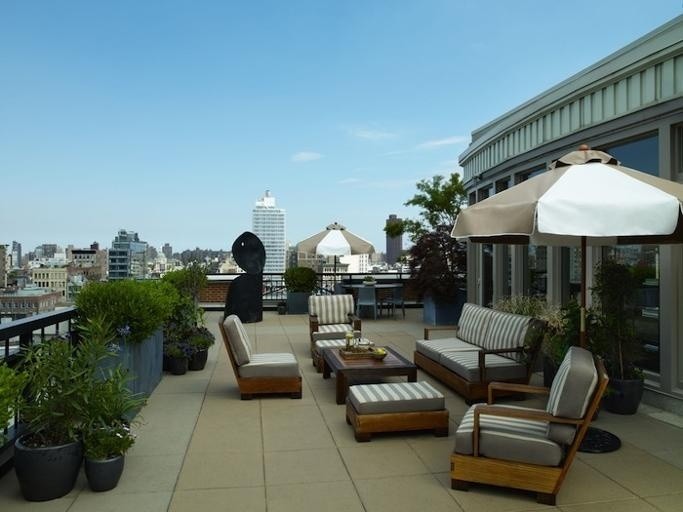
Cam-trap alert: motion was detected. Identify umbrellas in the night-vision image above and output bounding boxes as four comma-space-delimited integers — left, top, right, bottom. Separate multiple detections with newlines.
450, 144, 683, 453
295, 220, 377, 296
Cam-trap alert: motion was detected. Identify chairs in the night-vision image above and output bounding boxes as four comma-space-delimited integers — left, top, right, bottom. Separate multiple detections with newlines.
381, 289, 408, 320
357, 287, 378, 320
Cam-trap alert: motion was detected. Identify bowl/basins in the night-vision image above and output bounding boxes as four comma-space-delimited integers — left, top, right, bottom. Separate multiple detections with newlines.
371, 351, 387, 360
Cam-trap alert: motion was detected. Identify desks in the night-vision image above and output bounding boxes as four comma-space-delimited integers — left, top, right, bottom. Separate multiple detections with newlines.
342, 284, 403, 289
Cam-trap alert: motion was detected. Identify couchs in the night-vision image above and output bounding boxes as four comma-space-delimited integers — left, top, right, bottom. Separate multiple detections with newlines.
413, 301, 547, 405
217, 314, 302, 400
450, 346, 609, 504
308, 294, 361, 340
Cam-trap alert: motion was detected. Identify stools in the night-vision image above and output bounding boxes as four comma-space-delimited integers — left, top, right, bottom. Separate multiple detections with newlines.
344, 381, 449, 442
312, 337, 375, 372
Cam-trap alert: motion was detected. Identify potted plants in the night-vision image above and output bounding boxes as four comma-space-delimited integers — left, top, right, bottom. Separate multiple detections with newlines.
542, 308, 578, 385
12, 341, 88, 502
168, 344, 188, 375
363, 275, 377, 285
281, 267, 317, 314
590, 255, 645, 417
68, 418, 136, 492
184, 327, 216, 370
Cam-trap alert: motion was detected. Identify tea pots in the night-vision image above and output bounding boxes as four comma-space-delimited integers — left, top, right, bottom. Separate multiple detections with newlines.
354, 343, 374, 351
